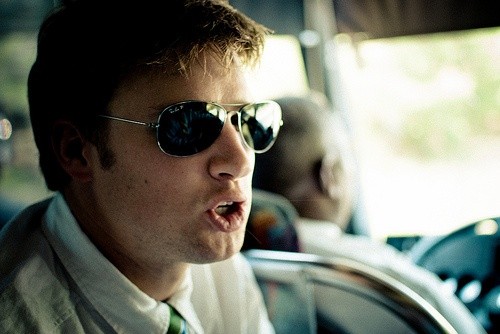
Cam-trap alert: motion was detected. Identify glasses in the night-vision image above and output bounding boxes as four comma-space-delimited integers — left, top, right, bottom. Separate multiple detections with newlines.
93, 100, 283, 158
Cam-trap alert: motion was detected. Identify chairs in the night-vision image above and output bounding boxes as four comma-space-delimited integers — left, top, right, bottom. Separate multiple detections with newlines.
243, 190, 461, 334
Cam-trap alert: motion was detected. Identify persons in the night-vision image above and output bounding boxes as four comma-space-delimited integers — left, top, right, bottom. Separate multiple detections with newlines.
250, 94, 487, 334
1, 0, 280, 334
